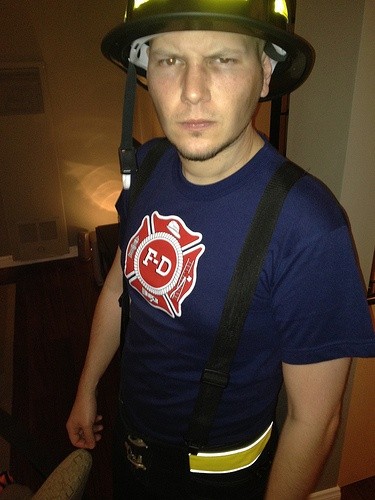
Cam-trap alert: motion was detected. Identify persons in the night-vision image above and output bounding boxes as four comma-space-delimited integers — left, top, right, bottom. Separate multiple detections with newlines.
66, 0, 375, 500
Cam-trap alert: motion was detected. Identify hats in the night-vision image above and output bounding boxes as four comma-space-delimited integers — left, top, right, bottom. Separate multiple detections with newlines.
101, 0, 314, 105
